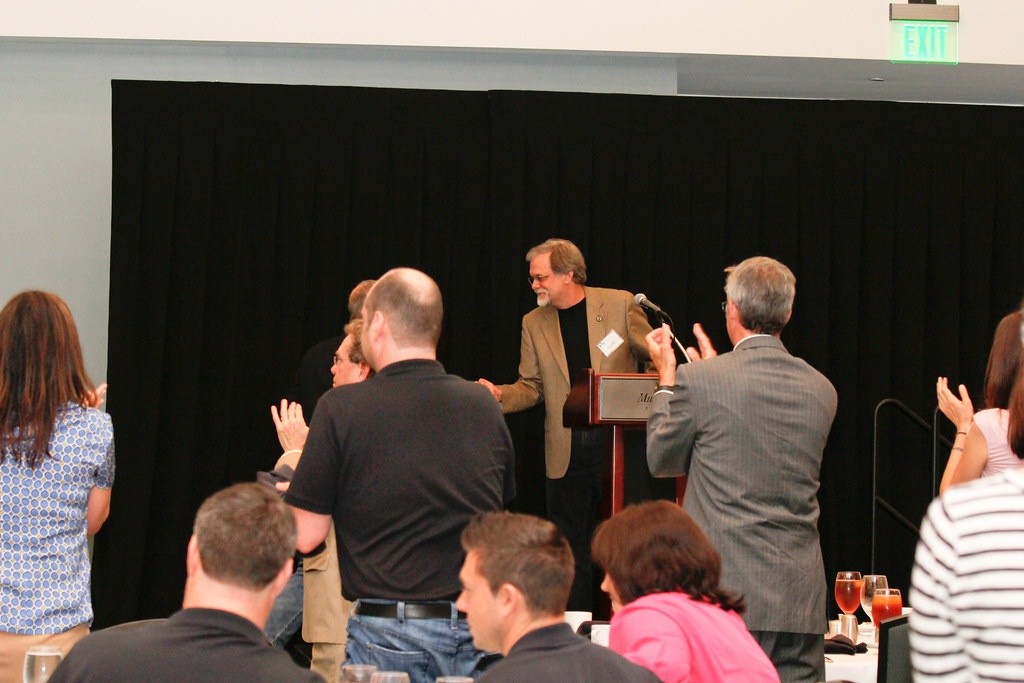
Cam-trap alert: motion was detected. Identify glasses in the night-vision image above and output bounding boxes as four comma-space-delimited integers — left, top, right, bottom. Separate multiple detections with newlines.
334, 356, 352, 366
527, 274, 549, 283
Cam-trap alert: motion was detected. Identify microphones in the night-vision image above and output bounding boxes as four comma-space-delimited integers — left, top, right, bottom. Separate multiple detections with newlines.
634, 293, 661, 313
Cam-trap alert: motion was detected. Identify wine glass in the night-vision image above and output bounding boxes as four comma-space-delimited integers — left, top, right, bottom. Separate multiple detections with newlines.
860, 575, 888, 647
835, 571, 862, 616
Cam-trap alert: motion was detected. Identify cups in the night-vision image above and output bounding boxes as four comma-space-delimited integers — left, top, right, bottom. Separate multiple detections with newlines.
22, 645, 63, 683
369, 671, 409, 683
590, 624, 610, 646
564, 611, 592, 633
435, 676, 474, 683
872, 588, 902, 630
340, 664, 375, 683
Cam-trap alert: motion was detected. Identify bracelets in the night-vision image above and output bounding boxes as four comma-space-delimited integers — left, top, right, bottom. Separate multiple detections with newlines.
654, 385, 674, 393
955, 432, 967, 436
280, 449, 304, 456
952, 446, 964, 452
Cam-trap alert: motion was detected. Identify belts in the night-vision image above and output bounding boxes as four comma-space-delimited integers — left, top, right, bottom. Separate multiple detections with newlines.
355, 600, 466, 618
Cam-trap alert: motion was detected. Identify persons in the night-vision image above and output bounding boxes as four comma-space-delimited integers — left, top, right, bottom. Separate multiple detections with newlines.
256, 267, 519, 683
474, 239, 659, 620
47, 481, 327, 683
908, 308, 1024, 683
591, 499, 781, 683
644, 256, 838, 683
456, 510, 663, 683
0, 288, 117, 683
